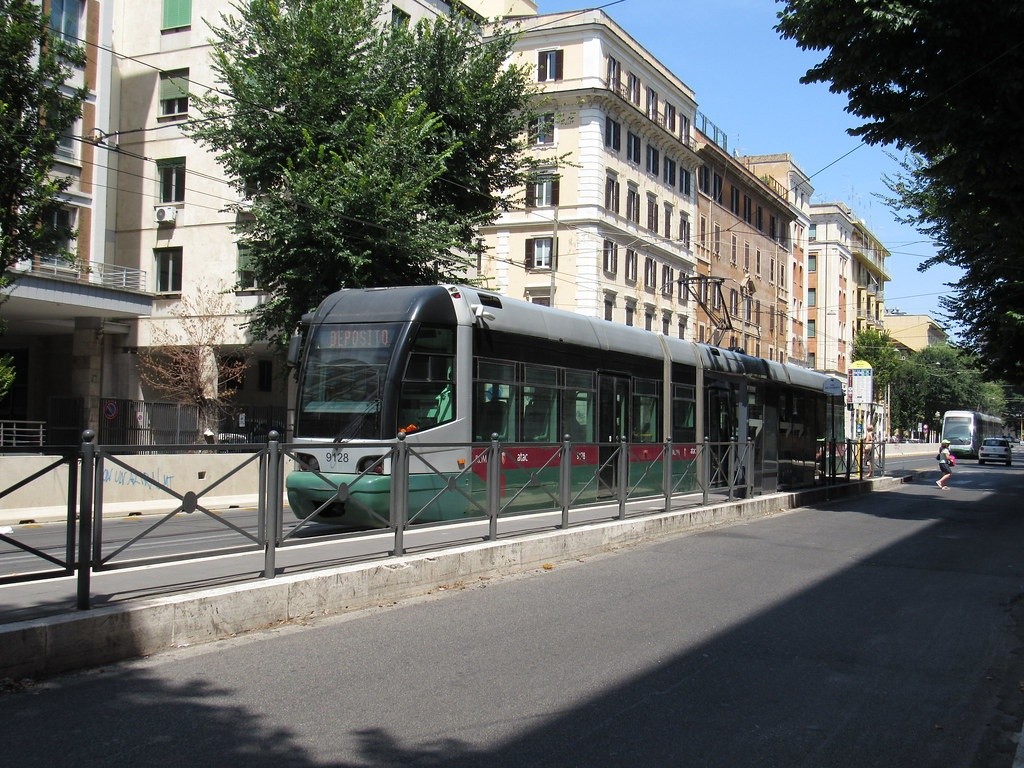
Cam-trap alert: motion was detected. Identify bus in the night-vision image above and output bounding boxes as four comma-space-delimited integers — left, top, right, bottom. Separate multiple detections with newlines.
940, 410, 1003, 457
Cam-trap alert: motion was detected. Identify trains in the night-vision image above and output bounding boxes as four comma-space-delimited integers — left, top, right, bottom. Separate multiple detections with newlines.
284, 285, 845, 525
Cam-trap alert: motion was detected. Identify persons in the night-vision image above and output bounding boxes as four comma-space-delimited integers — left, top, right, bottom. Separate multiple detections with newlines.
936, 439, 954, 490
864, 426, 875, 478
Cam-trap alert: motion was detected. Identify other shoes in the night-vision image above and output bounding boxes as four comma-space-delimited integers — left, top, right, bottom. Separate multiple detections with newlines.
942, 486, 950, 490
936, 480, 942, 487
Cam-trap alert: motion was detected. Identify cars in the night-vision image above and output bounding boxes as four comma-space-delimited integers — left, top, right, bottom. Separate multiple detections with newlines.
978, 438, 1013, 466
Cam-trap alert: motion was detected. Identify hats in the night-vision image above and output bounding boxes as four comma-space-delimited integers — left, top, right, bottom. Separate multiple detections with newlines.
941, 439, 950, 443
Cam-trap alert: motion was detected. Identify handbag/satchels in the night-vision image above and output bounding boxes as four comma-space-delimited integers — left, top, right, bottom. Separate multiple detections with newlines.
936, 454, 940, 460
